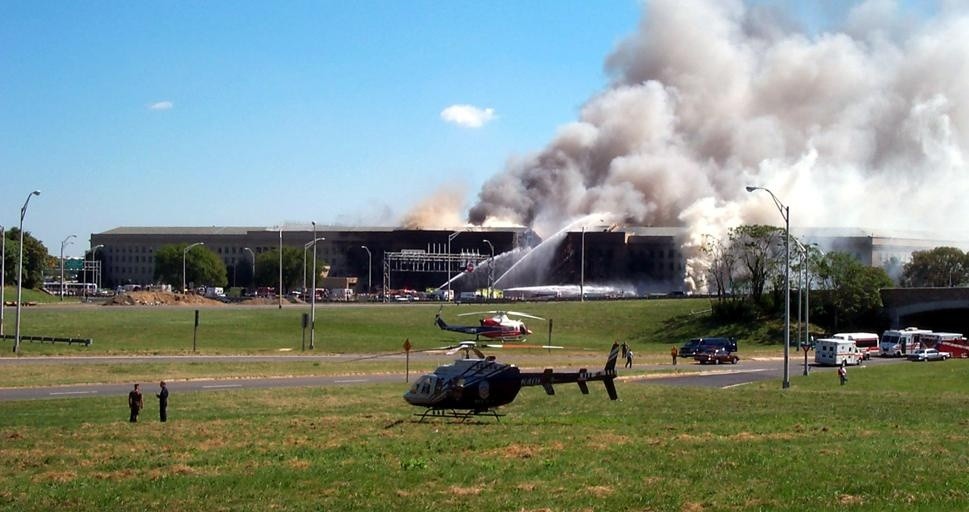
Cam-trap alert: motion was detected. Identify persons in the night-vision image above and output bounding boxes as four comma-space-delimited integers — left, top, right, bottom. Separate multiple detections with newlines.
625, 349, 633, 369
156, 381, 169, 422
621, 341, 628, 358
838, 365, 846, 385
671, 345, 678, 364
129, 382, 144, 422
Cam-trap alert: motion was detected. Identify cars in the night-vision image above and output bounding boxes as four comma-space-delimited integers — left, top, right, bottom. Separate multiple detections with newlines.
96, 285, 126, 297
907, 348, 950, 362
175, 284, 206, 296
666, 290, 685, 298
244, 291, 255, 297
729, 337, 737, 352
694, 347, 740, 364
257, 287, 276, 299
287, 287, 431, 302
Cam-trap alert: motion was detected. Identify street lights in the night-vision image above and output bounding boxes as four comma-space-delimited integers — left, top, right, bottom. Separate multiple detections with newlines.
82, 244, 104, 302
747, 187, 809, 388
304, 237, 325, 303
234, 259, 238, 287
483, 240, 494, 299
362, 245, 372, 297
309, 221, 316, 349
279, 224, 287, 308
184, 242, 204, 295
61, 235, 76, 301
581, 219, 604, 301
244, 247, 255, 289
13, 191, 40, 353
448, 227, 467, 304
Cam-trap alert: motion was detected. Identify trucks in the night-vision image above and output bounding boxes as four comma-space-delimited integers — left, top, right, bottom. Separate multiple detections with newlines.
815, 338, 864, 366
224, 286, 248, 298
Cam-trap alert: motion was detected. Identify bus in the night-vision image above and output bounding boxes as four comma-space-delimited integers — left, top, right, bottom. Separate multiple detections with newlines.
206, 286, 224, 297
832, 332, 881, 358
41, 282, 97, 296
124, 284, 172, 293
880, 327, 963, 358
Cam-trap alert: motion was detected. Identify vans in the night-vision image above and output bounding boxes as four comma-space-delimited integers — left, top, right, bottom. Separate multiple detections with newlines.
679, 335, 732, 358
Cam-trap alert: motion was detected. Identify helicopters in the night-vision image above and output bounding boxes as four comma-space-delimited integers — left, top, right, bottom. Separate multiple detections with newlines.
433, 302, 546, 343
402, 341, 619, 424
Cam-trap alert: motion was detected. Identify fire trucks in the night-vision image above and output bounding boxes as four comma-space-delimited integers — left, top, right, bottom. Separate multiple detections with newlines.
461, 287, 504, 299
424, 287, 454, 301
